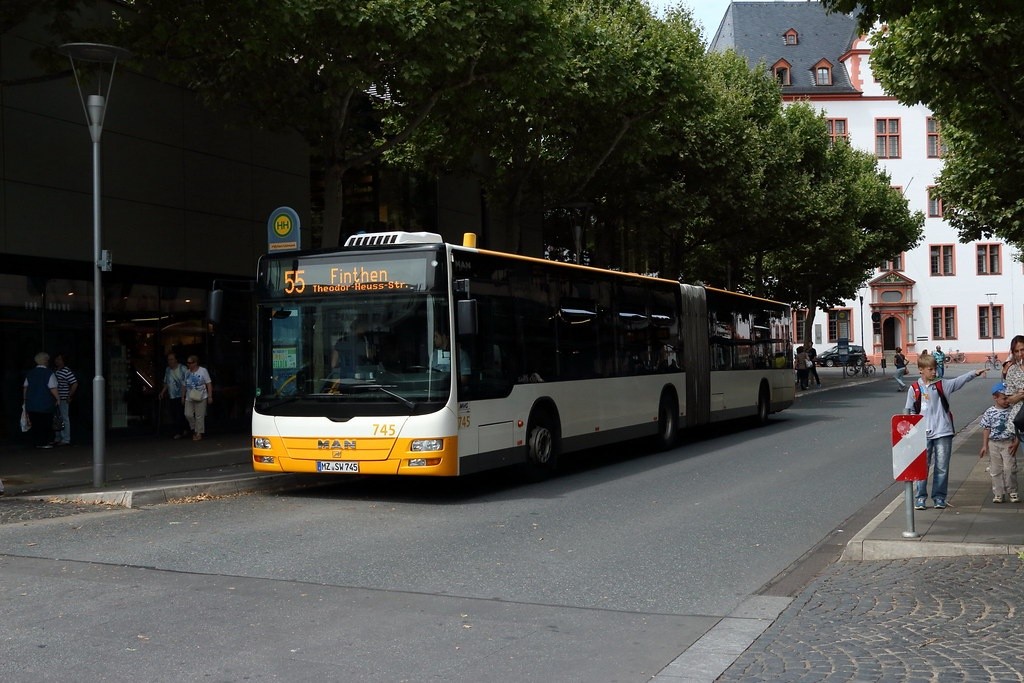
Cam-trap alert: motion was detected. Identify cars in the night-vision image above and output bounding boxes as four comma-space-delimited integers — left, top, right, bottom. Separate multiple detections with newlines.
817, 345, 867, 368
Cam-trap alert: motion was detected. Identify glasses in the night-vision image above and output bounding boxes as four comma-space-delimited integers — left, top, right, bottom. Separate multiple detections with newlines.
187, 362, 194, 364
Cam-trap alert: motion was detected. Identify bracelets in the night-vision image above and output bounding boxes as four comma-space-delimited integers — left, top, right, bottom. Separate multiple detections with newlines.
56, 397, 61, 400
69, 392, 73, 397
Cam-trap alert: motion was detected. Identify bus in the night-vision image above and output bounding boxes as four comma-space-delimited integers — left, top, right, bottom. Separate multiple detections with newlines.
208, 206, 795, 486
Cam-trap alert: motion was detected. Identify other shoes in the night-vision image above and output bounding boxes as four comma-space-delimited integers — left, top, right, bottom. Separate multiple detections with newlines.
174, 434, 182, 440
192, 432, 203, 440
35, 440, 66, 449
182, 430, 187, 436
898, 385, 907, 392
806, 386, 810, 390
817, 384, 822, 388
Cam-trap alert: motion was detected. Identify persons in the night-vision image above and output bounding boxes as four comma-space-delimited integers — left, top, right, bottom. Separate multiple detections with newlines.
425, 326, 482, 398
158, 351, 213, 441
893, 347, 909, 392
22, 351, 79, 449
796, 340, 822, 390
933, 345, 948, 378
330, 318, 373, 368
906, 354, 991, 509
919, 350, 927, 377
980, 335, 1024, 503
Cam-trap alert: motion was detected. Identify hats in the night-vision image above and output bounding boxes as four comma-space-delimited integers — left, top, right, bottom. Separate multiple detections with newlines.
992, 382, 1005, 394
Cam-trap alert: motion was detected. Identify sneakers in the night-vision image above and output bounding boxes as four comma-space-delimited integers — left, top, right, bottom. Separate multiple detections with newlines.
993, 494, 1006, 503
1009, 492, 1022, 503
914, 496, 926, 510
934, 497, 946, 508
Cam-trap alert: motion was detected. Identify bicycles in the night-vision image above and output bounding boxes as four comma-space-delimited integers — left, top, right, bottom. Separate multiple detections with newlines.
846, 356, 876, 378
985, 354, 1002, 371
944, 350, 968, 364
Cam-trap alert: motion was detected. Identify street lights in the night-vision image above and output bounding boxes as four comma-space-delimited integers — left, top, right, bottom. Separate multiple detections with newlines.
57, 42, 131, 491
985, 292, 998, 362
858, 285, 867, 375
564, 201, 597, 264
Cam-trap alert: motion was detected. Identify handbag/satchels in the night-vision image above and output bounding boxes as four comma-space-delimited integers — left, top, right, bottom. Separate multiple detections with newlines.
20, 404, 32, 432
805, 353, 813, 368
1013, 402, 1024, 442
189, 389, 202, 401
53, 403, 65, 431
904, 368, 909, 375
